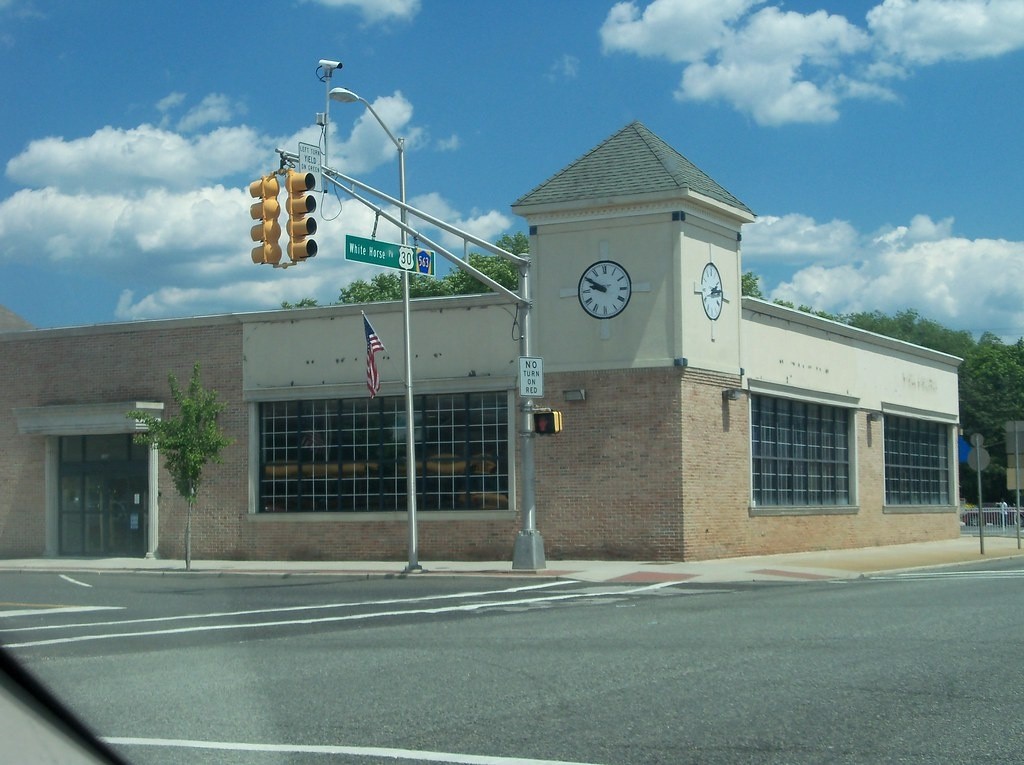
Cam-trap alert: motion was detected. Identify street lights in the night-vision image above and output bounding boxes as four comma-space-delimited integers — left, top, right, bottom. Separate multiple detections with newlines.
329, 88, 421, 573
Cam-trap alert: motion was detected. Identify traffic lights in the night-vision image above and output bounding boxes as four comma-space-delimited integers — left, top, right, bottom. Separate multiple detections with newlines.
250, 176, 282, 264
534, 412, 559, 435
285, 170, 319, 262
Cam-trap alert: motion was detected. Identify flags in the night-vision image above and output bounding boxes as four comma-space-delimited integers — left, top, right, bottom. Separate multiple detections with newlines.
363, 317, 386, 399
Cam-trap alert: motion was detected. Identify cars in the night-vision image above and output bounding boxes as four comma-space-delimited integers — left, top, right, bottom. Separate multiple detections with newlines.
963, 503, 1024, 528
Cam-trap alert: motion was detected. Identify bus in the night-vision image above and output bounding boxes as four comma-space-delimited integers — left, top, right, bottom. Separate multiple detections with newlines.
264, 454, 507, 509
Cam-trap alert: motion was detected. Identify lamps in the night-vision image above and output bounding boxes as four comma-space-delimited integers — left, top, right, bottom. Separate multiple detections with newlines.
562, 389, 585, 401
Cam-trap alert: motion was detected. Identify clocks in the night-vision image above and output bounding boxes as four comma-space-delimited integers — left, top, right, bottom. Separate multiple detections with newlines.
700, 261, 723, 320
578, 259, 632, 319
